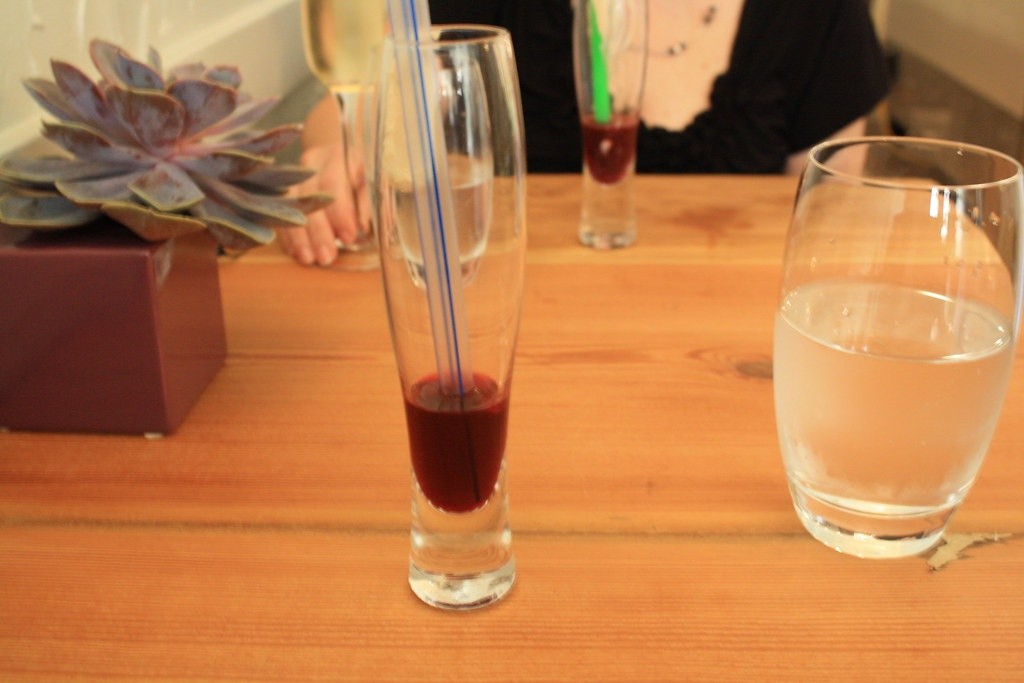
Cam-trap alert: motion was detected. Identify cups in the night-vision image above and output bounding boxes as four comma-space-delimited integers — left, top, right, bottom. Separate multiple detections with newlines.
772, 137, 1024, 560
372, 23, 528, 614
570, 1, 650, 248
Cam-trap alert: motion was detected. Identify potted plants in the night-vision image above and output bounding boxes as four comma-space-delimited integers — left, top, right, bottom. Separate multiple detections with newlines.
1, 50, 322, 432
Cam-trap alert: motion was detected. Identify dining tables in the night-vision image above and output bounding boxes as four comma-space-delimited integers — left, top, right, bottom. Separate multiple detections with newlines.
0, 156, 1024, 683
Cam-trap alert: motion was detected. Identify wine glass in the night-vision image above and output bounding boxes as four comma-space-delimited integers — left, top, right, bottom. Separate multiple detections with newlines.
297, 0, 390, 272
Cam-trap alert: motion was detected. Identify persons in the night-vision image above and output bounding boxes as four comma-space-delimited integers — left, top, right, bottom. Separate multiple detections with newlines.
276, 1, 896, 270
